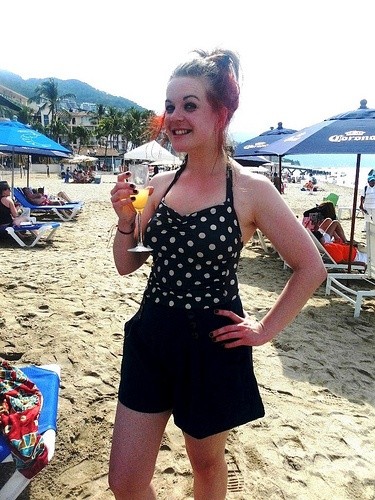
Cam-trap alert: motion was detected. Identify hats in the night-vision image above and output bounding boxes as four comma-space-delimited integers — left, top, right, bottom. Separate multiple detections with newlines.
368, 169, 375, 182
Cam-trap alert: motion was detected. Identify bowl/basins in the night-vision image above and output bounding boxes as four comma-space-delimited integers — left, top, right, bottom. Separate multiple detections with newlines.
324, 193, 339, 206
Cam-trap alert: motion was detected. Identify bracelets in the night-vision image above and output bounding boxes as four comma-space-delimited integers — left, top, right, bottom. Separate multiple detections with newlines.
116, 224, 136, 234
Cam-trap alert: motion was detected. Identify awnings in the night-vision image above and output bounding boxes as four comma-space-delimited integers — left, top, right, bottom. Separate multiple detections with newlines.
78, 146, 118, 156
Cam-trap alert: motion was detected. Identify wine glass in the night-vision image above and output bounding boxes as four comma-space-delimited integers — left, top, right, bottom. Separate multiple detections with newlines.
127, 165, 154, 252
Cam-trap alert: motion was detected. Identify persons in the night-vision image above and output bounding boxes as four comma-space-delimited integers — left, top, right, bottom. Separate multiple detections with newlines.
60, 162, 106, 184
109, 49, 329, 500
283, 170, 318, 192
46, 164, 49, 177
301, 202, 367, 265
0, 181, 47, 239
272, 172, 283, 194
359, 176, 375, 232
22, 187, 79, 211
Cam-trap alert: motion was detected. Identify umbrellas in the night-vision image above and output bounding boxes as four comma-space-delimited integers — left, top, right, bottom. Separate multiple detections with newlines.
0, 115, 72, 201
234, 122, 299, 194
254, 99, 375, 294
123, 140, 183, 182
232, 156, 271, 175
62, 155, 98, 172
0, 144, 73, 188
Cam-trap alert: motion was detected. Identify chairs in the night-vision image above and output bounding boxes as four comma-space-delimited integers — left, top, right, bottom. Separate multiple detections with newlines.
1, 362, 59, 500
9, 187, 85, 222
0, 223, 60, 248
249, 222, 375, 318
